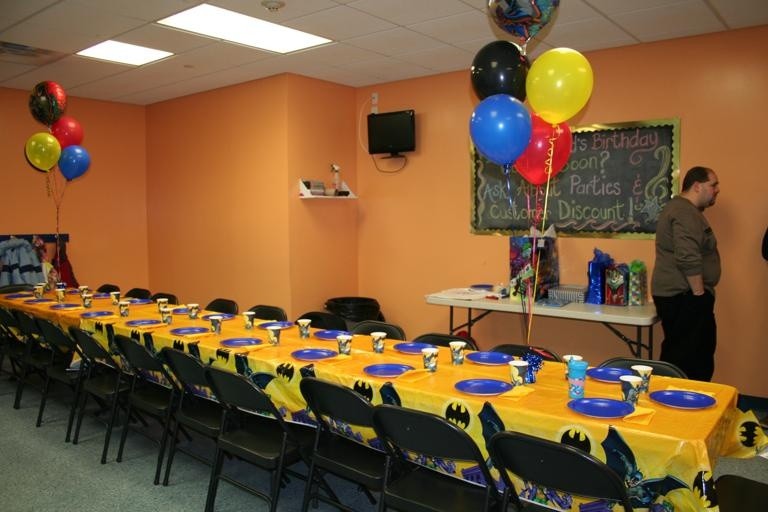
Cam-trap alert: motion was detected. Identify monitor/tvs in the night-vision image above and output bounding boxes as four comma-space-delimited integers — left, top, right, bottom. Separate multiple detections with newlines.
367, 109, 416, 158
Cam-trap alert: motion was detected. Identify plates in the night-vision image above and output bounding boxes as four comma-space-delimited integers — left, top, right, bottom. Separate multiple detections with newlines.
363, 364, 415, 380
49, 303, 81, 310
467, 351, 515, 367
645, 390, 717, 410
126, 319, 162, 327
291, 348, 338, 359
171, 309, 199, 314
127, 298, 153, 304
80, 311, 114, 319
258, 321, 295, 330
314, 330, 349, 341
92, 293, 111, 299
170, 327, 208, 335
220, 337, 262, 348
565, 396, 636, 421
453, 377, 510, 398
202, 314, 233, 321
24, 298, 53, 304
5, 293, 32, 300
394, 340, 440, 355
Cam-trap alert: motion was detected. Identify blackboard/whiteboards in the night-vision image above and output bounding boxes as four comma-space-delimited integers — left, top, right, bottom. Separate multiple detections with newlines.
469, 116, 682, 241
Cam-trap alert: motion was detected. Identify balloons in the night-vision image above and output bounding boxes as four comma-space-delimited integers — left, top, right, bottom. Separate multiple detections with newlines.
470, 0, 595, 186
25, 80, 93, 180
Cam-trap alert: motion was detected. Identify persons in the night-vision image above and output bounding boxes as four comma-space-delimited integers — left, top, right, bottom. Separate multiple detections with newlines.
650, 166, 721, 381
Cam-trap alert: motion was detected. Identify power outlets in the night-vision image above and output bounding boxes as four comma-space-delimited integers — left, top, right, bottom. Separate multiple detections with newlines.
372, 92, 378, 104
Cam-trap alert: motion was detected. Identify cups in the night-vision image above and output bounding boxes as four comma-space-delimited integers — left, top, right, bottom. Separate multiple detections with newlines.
119, 302, 130, 317
370, 332, 387, 354
56, 289, 66, 304
78, 286, 89, 297
337, 335, 353, 358
161, 309, 173, 325
187, 304, 200, 319
266, 327, 281, 346
242, 311, 256, 329
81, 294, 93, 308
297, 318, 312, 341
208, 316, 223, 335
33, 286, 44, 298
57, 282, 67, 295
111, 292, 120, 305
616, 364, 652, 407
156, 298, 168, 313
506, 360, 528, 391
564, 355, 583, 398
423, 338, 467, 375
